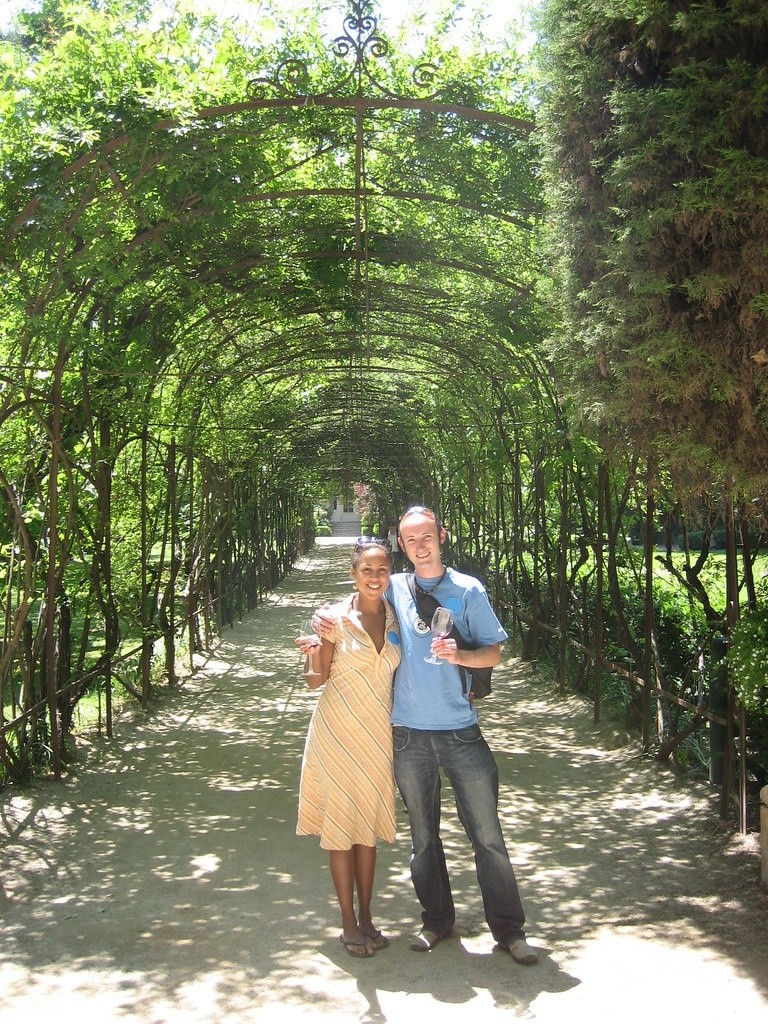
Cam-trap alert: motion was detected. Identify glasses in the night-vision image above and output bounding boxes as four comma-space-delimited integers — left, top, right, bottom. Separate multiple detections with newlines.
398, 506, 435, 521
355, 536, 392, 553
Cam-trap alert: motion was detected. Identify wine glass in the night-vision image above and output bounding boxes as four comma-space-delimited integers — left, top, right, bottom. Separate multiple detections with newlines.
424, 608, 454, 665
300, 619, 321, 676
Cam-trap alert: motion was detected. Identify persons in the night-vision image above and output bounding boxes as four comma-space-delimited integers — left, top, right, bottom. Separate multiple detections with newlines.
295, 537, 401, 958
310, 505, 536, 964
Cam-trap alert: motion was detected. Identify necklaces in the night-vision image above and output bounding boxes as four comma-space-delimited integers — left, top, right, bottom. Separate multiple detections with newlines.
414, 565, 447, 594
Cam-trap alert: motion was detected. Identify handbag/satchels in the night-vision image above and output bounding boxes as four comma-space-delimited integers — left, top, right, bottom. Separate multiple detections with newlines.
406, 573, 493, 699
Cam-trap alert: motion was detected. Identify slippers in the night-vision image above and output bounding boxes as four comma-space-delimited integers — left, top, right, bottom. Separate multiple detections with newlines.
364, 931, 389, 950
340, 934, 374, 957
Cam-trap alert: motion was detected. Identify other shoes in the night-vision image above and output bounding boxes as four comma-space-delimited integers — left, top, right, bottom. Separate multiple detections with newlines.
498, 938, 537, 964
410, 930, 443, 951
